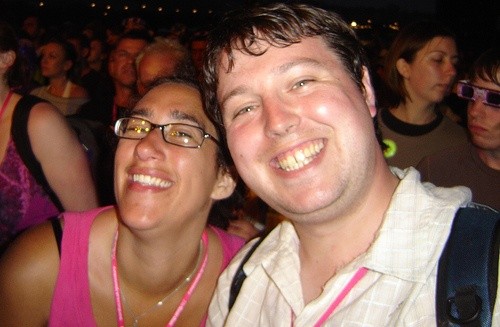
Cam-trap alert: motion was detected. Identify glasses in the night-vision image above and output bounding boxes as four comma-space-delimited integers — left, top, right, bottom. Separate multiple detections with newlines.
456, 81, 500, 108
113, 117, 220, 149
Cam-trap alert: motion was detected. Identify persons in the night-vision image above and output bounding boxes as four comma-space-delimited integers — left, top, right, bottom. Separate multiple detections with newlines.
202, 4, 500, 327
415, 55, 500, 214
0, 23, 98, 256
372, 23, 468, 170
1, 71, 248, 327
5, 13, 216, 208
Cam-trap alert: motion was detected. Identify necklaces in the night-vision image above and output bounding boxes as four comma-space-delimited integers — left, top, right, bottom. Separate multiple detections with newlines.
119, 239, 203, 327
112, 228, 210, 326
